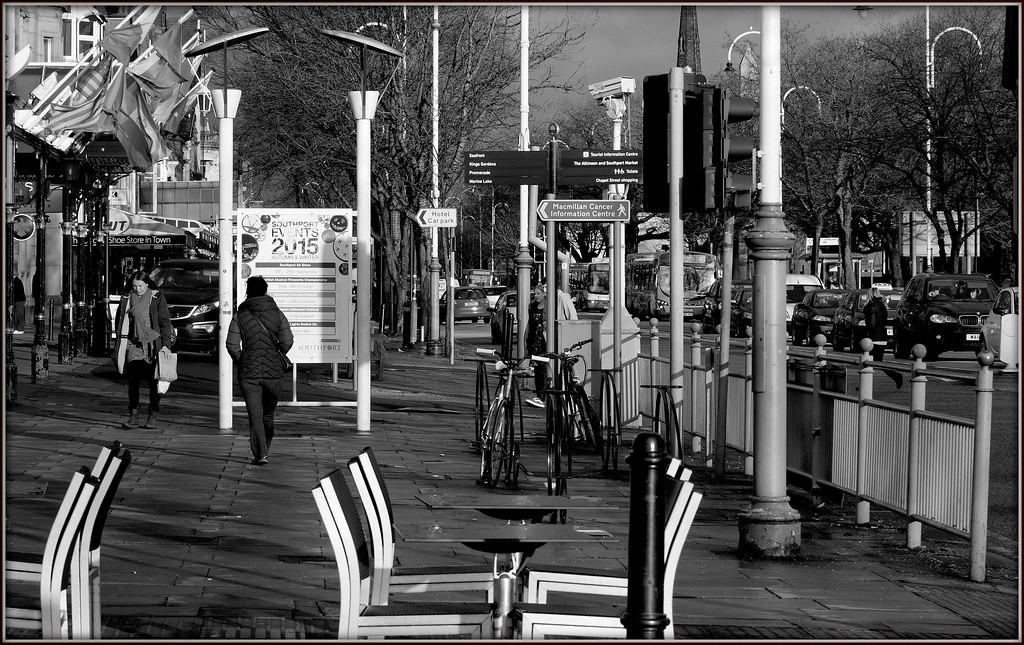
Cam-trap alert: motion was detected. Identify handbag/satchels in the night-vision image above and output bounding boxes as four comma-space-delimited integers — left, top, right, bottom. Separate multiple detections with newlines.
154, 352, 177, 382
279, 351, 292, 371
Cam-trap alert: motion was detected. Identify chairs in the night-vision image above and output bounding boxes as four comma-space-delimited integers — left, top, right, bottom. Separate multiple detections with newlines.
3, 439, 133, 640
312, 446, 702, 645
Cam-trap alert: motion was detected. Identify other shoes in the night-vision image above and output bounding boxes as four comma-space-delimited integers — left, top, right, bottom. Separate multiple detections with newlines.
146, 410, 158, 428
122, 409, 139, 428
252, 455, 268, 463
896, 373, 903, 389
13, 330, 24, 334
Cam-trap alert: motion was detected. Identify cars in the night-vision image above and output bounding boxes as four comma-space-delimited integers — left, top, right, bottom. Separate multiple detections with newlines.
138, 260, 238, 361
440, 250, 1020, 366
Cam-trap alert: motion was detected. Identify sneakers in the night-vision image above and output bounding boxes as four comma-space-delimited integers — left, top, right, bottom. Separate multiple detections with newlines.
525, 398, 545, 407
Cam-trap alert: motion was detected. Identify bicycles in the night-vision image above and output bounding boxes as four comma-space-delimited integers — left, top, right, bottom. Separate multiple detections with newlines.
533, 334, 601, 456
475, 347, 550, 488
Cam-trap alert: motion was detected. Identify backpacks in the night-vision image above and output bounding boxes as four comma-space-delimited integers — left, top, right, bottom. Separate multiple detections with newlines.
867, 297, 887, 330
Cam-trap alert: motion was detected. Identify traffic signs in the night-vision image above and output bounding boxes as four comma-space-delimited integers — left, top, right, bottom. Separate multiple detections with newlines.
415, 208, 457, 227
537, 199, 631, 220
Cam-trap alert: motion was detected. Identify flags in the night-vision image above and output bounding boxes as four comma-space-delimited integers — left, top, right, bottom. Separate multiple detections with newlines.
42, 5, 218, 170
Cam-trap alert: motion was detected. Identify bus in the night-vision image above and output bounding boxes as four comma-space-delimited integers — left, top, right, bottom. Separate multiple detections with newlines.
96, 221, 237, 339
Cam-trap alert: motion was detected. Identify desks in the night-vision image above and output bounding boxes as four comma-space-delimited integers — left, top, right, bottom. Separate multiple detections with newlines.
392, 522, 620, 641
414, 494, 619, 607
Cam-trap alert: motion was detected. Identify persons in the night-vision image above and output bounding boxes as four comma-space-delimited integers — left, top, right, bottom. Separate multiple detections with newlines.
225, 274, 293, 465
111, 271, 172, 429
10, 275, 26, 334
522, 276, 578, 409
967, 288, 982, 301
861, 287, 889, 362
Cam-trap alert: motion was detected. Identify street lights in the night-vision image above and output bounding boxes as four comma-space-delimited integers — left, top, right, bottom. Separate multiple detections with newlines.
318, 28, 405, 430
184, 25, 271, 428
443, 7, 989, 270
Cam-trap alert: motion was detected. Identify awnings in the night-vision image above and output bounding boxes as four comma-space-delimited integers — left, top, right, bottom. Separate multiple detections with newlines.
70, 196, 196, 252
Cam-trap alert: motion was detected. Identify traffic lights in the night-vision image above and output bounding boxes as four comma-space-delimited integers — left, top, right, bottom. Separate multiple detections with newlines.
724, 90, 756, 208
